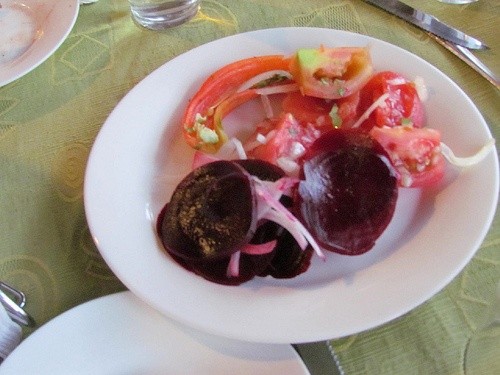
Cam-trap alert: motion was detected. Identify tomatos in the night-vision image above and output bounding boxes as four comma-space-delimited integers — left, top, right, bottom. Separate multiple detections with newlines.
283, 43, 444, 188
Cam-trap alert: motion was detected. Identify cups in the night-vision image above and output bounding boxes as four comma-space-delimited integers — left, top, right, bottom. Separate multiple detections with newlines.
127, 0, 198, 31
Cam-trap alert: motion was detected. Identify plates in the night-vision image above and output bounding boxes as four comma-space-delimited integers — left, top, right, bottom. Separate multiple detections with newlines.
0, 291, 311, 375
0, 0, 80, 88
83, 27, 500, 345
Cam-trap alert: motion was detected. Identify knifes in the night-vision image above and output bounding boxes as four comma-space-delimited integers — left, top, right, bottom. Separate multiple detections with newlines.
361, 0, 490, 52
